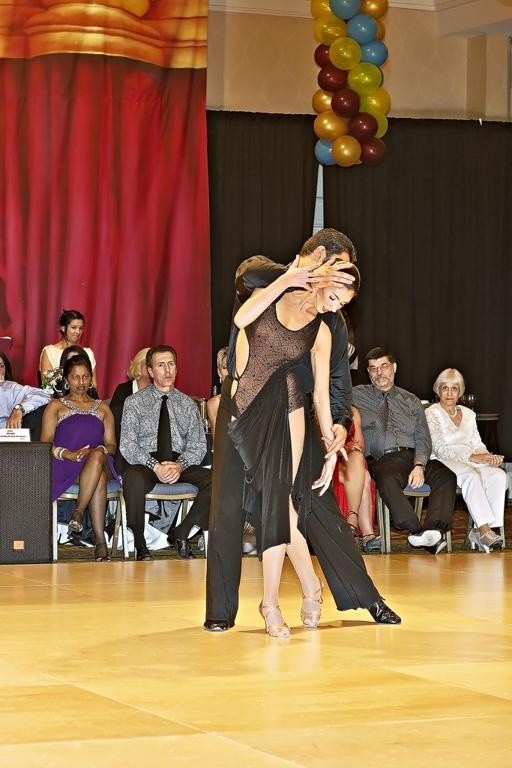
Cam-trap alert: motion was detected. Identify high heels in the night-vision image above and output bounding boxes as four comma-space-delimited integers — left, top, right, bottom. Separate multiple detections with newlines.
259, 600, 291, 637
95, 543, 111, 562
468, 528, 503, 553
362, 534, 383, 555
346, 511, 358, 531
301, 576, 325, 628
68, 509, 84, 534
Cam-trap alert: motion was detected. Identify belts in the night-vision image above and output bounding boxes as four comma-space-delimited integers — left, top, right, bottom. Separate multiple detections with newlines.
384, 446, 415, 455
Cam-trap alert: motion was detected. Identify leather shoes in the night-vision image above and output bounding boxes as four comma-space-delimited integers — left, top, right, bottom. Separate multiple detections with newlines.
368, 599, 401, 624
408, 529, 447, 555
204, 620, 234, 632
137, 546, 152, 560
167, 530, 197, 559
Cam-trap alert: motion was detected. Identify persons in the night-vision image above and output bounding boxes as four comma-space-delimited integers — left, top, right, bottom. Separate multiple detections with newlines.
227, 254, 362, 638
37, 308, 227, 563
332, 406, 383, 553
204, 228, 402, 632
424, 368, 507, 553
0, 352, 51, 428
352, 347, 457, 554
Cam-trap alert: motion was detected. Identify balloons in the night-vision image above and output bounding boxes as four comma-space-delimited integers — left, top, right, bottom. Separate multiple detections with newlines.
311, 0, 391, 168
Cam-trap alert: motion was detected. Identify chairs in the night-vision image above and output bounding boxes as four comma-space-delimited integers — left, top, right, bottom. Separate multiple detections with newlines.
52, 479, 128, 562
134, 483, 209, 561
446, 487, 505, 553
376, 482, 445, 554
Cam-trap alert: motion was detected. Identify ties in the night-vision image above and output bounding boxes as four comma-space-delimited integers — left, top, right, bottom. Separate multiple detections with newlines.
370, 392, 389, 461
157, 395, 173, 461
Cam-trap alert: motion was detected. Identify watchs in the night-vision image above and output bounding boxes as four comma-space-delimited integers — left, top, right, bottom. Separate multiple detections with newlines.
14, 404, 25, 414
415, 463, 426, 471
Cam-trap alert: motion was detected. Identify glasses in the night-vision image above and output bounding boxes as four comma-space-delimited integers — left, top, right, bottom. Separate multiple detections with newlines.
368, 363, 392, 372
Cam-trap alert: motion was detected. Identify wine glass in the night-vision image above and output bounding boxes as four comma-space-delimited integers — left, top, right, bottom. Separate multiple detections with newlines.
466, 394, 477, 411
457, 395, 466, 406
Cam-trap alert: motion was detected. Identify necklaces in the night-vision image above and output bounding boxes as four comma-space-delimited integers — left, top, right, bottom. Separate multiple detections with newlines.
448, 408, 458, 419
